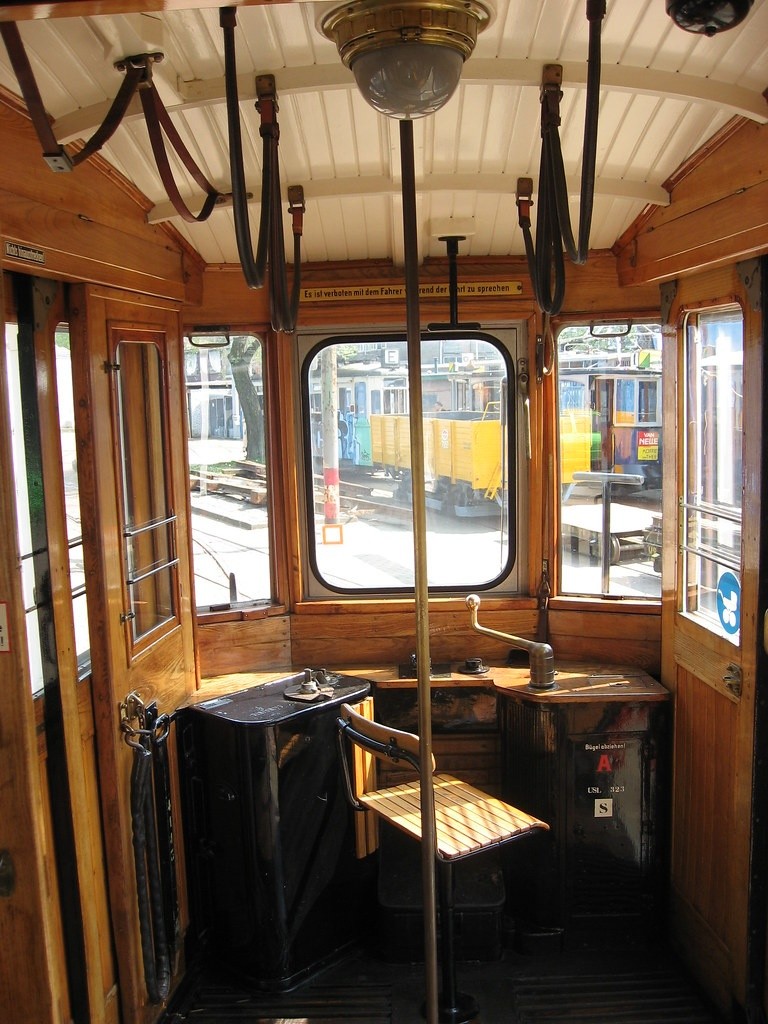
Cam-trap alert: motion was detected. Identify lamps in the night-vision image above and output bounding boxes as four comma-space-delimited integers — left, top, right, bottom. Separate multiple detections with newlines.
312, 0, 497, 121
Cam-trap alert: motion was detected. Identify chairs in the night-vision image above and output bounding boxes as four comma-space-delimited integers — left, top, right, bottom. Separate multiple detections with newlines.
337, 703, 551, 1024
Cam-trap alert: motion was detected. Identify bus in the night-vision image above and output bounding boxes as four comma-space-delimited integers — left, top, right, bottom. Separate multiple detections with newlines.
180, 351, 662, 519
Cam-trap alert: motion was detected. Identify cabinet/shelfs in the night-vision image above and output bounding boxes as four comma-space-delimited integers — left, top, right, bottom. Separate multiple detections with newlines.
334, 667, 668, 924
175, 672, 377, 995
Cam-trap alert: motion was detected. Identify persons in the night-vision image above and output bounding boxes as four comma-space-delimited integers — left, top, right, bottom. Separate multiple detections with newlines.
435, 402, 445, 412
